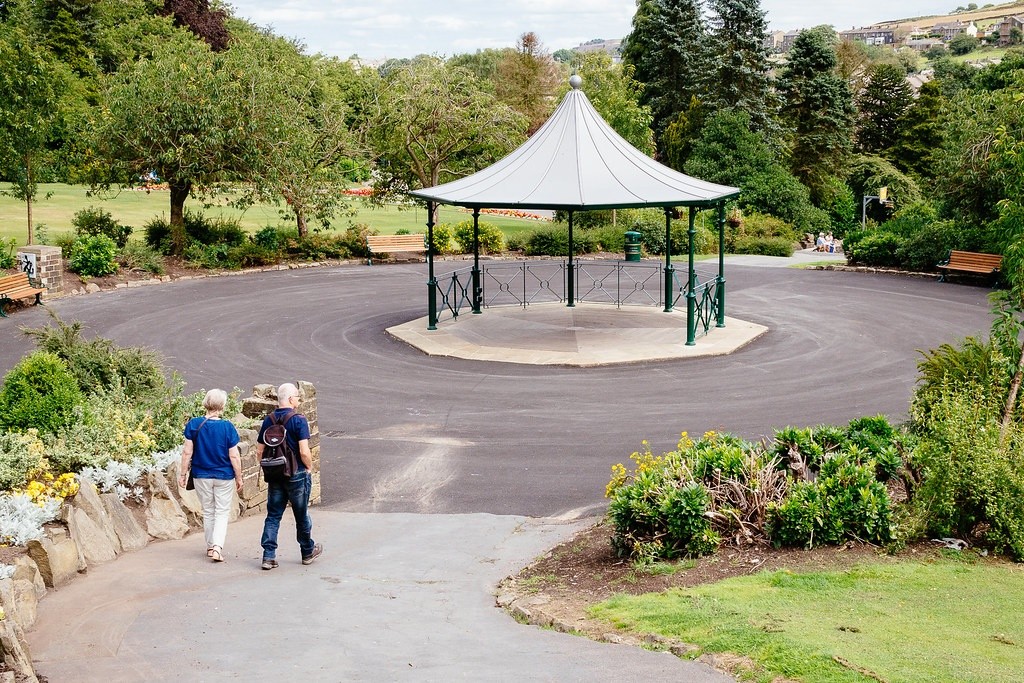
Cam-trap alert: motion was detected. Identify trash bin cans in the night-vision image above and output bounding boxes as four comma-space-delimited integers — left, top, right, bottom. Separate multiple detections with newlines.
623, 232, 642, 262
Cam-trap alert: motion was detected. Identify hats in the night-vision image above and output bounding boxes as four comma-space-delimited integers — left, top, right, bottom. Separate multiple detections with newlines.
819, 232, 824, 236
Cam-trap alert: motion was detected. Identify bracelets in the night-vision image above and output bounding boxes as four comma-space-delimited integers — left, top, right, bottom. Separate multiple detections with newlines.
181, 474, 186, 476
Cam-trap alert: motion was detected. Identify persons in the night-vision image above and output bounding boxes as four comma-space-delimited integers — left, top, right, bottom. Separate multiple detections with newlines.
179, 389, 244, 562
257, 382, 324, 570
817, 232, 828, 253
824, 232, 833, 254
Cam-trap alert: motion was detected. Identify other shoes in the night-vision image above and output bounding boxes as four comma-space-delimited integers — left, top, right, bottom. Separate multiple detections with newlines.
212, 550, 223, 561
207, 548, 213, 557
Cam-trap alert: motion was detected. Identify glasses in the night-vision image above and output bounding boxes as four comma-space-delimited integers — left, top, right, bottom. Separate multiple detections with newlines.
294, 395, 301, 398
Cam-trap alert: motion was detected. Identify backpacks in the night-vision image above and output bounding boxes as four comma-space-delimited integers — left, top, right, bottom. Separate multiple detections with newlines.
260, 412, 302, 480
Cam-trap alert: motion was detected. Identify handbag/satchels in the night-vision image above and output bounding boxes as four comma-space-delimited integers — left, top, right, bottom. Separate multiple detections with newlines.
186, 468, 194, 490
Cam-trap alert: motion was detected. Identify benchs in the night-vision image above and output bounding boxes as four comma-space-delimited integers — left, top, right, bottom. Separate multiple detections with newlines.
936, 249, 1005, 290
366, 234, 430, 266
0, 272, 50, 317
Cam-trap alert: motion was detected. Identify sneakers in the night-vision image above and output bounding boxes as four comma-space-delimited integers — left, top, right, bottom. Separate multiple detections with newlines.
261, 559, 279, 569
302, 543, 323, 564
